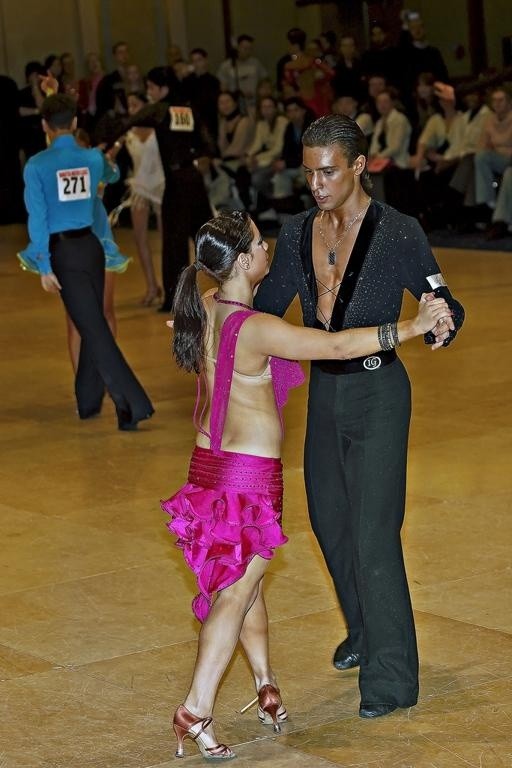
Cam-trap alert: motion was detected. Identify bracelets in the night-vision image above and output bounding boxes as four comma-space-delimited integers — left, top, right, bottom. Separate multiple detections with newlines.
376, 319, 400, 353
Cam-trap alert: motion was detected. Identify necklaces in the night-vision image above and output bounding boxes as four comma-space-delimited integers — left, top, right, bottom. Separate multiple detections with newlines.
319, 196, 372, 265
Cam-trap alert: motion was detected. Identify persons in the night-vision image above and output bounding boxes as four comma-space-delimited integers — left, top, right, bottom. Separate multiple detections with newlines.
0, 4, 511, 238
111, 65, 215, 315
17, 68, 135, 382
160, 209, 455, 761
166, 113, 466, 720
105, 92, 164, 305
19, 93, 155, 432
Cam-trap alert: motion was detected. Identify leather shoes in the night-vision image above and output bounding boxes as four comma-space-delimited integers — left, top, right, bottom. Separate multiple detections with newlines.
333, 637, 360, 670
359, 703, 397, 718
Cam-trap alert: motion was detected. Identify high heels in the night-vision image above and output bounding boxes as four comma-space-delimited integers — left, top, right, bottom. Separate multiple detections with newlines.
257, 685, 288, 733
142, 288, 162, 307
173, 704, 235, 760
